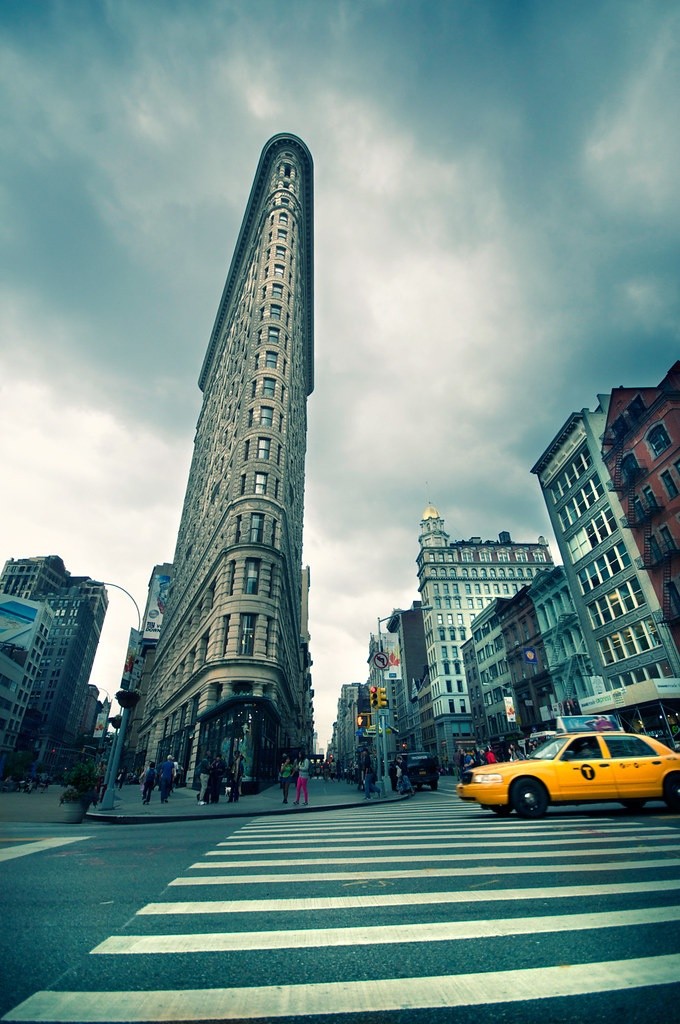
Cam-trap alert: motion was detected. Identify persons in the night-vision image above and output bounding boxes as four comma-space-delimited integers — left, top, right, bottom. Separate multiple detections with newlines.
5, 774, 50, 794
98, 763, 107, 793
309, 759, 336, 784
453, 741, 525, 781
390, 757, 402, 792
128, 774, 133, 780
525, 739, 537, 754
361, 749, 381, 800
116, 769, 124, 790
138, 755, 184, 805
198, 750, 244, 805
279, 750, 309, 805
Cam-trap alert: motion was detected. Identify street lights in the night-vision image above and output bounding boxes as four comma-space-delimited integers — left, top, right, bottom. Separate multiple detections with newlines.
84, 579, 141, 811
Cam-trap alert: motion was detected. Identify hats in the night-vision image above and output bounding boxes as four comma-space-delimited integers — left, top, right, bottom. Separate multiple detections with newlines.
361, 747, 368, 753
166, 755, 174, 760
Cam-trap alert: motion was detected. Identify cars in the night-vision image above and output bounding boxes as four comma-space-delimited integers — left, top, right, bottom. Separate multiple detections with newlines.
455, 715, 680, 820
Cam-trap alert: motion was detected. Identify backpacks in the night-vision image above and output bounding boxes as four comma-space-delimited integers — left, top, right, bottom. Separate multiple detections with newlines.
465, 755, 478, 768
145, 768, 155, 787
194, 761, 202, 778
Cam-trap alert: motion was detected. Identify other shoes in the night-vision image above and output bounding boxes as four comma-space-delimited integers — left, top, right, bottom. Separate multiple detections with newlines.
164, 799, 168, 803
411, 791, 416, 796
302, 801, 308, 805
293, 801, 299, 805
198, 801, 207, 806
378, 790, 382, 798
143, 799, 147, 805
363, 797, 372, 802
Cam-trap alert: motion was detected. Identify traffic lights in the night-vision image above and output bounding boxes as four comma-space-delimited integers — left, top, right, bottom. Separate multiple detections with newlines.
402, 744, 406, 747
369, 687, 379, 709
357, 715, 370, 727
379, 688, 388, 707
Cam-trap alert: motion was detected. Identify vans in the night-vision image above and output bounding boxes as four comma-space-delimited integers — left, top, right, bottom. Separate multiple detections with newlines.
398, 752, 440, 791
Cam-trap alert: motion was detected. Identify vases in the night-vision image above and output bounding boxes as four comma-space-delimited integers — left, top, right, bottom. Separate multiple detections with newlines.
118, 695, 138, 708
111, 721, 122, 728
61, 800, 83, 823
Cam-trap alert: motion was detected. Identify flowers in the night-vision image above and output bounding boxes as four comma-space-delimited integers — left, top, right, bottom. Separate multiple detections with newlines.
109, 715, 122, 722
115, 689, 144, 699
58, 786, 81, 803
